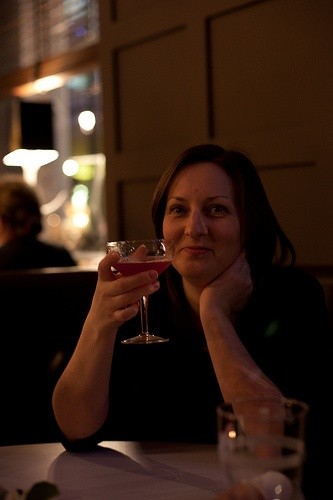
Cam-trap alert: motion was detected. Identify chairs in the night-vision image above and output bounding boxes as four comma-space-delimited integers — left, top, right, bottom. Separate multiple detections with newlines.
0, 267, 98, 441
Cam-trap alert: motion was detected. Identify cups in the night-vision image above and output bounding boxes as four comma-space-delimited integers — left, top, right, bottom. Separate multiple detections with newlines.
216, 397, 309, 500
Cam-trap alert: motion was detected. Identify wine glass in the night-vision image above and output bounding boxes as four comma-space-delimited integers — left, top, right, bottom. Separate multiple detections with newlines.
106, 240, 173, 345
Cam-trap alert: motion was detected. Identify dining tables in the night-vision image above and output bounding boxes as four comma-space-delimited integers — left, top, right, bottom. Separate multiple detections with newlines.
0, 444, 303, 499
